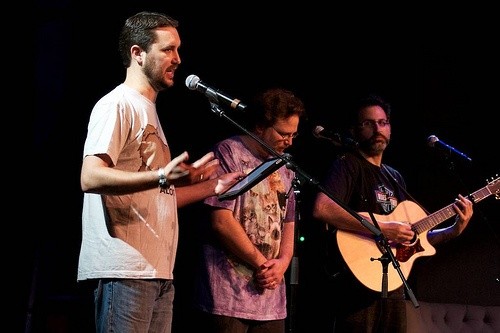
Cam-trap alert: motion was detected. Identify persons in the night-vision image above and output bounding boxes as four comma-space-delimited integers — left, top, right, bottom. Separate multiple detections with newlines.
205, 90, 304, 333
313, 96, 474, 333
77, 12, 247, 333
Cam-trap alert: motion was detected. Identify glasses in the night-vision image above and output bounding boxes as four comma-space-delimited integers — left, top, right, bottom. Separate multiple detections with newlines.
270, 126, 299, 140
357, 120, 390, 128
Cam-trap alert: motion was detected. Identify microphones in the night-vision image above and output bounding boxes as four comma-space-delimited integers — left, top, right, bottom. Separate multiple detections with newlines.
185, 74, 248, 112
313, 125, 359, 148
426, 135, 472, 162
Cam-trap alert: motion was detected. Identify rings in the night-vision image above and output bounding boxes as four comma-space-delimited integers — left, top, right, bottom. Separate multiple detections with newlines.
269, 280, 276, 287
200, 173, 204, 181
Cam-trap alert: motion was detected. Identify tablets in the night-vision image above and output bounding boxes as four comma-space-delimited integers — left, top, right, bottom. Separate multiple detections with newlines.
218, 152, 290, 200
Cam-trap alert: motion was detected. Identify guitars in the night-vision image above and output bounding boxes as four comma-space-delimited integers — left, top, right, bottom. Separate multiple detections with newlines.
329, 173, 500, 294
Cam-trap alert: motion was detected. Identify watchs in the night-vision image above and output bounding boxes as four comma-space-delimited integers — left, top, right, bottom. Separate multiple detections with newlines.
158, 167, 170, 189
